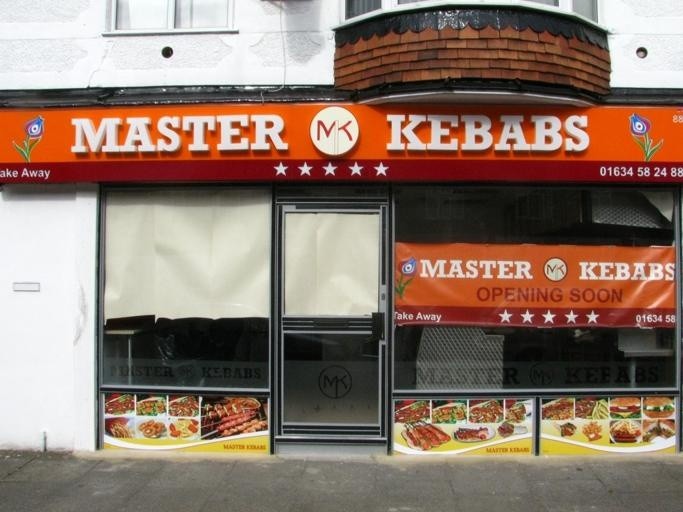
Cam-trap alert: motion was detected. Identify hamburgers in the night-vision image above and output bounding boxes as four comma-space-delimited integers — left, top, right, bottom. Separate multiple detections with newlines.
643, 397, 674, 417
609, 398, 641, 418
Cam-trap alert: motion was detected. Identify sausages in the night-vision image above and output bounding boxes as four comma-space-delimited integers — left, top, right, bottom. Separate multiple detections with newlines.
614, 434, 637, 442
217, 410, 256, 433
406, 423, 450, 450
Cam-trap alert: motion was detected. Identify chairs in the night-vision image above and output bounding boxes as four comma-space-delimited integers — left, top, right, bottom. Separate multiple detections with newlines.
618, 328, 674, 388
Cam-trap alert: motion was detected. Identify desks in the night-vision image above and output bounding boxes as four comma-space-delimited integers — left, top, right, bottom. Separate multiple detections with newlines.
103, 328, 148, 384
